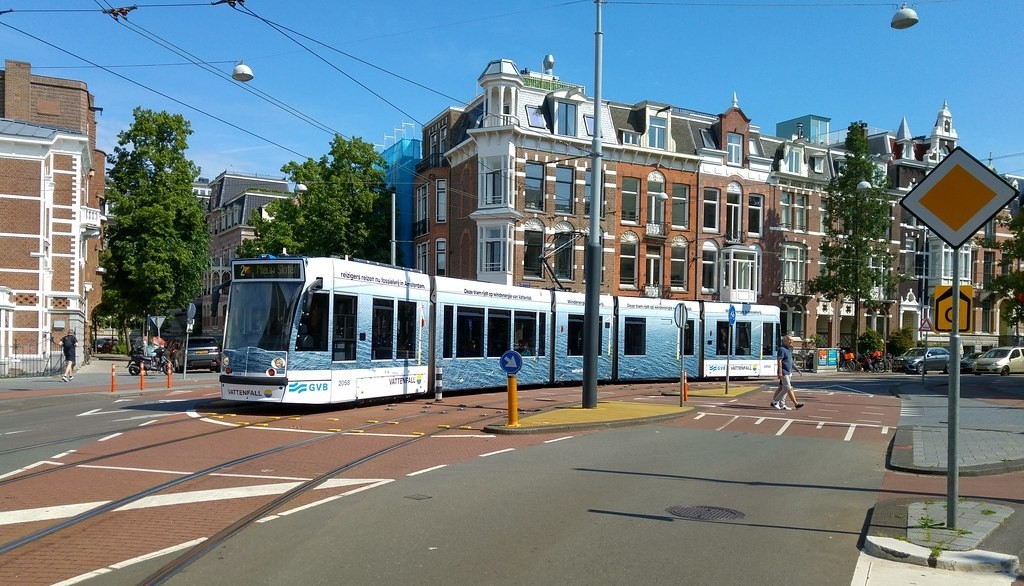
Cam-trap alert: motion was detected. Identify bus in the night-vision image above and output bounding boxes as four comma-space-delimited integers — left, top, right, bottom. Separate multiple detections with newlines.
211, 231, 785, 407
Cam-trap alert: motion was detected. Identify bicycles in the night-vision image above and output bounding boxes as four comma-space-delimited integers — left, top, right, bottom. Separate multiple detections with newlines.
791, 351, 813, 373
840, 348, 893, 374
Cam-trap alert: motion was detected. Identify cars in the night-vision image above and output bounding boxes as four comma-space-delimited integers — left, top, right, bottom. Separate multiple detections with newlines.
960, 351, 986, 374
892, 346, 950, 375
128, 328, 220, 373
972, 346, 1024, 377
92, 336, 119, 354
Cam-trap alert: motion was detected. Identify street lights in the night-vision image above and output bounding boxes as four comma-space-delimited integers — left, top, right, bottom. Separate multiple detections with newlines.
852, 173, 872, 358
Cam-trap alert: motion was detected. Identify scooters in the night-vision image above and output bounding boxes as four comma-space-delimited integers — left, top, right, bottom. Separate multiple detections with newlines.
126, 344, 175, 375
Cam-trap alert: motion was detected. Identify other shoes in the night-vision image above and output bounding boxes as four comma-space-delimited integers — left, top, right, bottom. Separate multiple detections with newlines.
770, 402, 774, 407
772, 399, 781, 410
795, 403, 804, 409
69, 376, 74, 381
780, 405, 792, 411
62, 377, 68, 382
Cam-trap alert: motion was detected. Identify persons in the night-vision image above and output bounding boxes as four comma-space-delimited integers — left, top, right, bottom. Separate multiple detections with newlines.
737, 346, 744, 354
770, 335, 805, 411
128, 332, 185, 373
720, 335, 726, 354
466, 338, 545, 357
764, 344, 771, 355
59, 329, 79, 383
